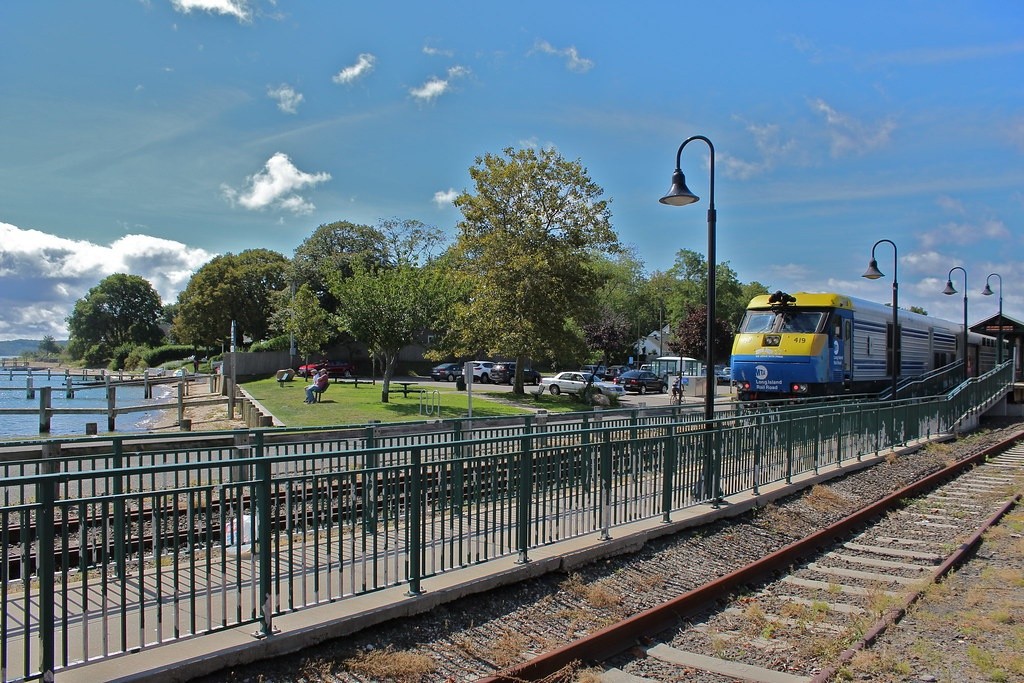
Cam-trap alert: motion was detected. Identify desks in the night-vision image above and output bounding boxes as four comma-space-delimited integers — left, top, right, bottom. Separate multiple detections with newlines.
394, 383, 418, 398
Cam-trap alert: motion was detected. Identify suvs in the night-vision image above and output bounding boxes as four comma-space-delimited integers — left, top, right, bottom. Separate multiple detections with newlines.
579, 364, 608, 379
461, 360, 495, 384
489, 361, 541, 386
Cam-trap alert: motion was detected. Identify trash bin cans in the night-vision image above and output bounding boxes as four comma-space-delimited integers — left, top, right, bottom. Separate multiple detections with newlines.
456, 376, 467, 392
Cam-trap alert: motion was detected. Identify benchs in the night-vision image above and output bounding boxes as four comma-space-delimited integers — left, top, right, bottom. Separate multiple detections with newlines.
389, 390, 425, 393
530, 384, 545, 401
277, 373, 289, 388
313, 382, 330, 403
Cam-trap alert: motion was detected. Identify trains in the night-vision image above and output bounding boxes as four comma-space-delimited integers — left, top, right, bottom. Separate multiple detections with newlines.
729, 289, 1012, 408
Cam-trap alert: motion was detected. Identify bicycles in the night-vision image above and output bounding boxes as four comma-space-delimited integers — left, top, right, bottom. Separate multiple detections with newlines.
669, 389, 686, 405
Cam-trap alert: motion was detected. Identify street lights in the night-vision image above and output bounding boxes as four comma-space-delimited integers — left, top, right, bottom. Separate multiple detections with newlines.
658, 134, 724, 500
940, 266, 968, 380
861, 238, 899, 400
981, 273, 1003, 366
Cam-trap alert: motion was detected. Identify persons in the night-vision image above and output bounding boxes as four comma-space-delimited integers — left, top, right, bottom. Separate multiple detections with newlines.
571, 374, 579, 381
617, 368, 623, 376
303, 369, 320, 402
306, 368, 328, 404
672, 376, 684, 402
610, 370, 615, 377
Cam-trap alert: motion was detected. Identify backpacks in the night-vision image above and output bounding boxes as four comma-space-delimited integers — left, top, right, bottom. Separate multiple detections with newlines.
673, 378, 679, 386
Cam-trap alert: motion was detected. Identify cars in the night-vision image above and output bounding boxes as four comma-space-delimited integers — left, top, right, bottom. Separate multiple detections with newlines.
614, 369, 668, 395
431, 362, 461, 383
298, 358, 359, 379
541, 371, 626, 398
640, 362, 731, 385
605, 364, 630, 381
